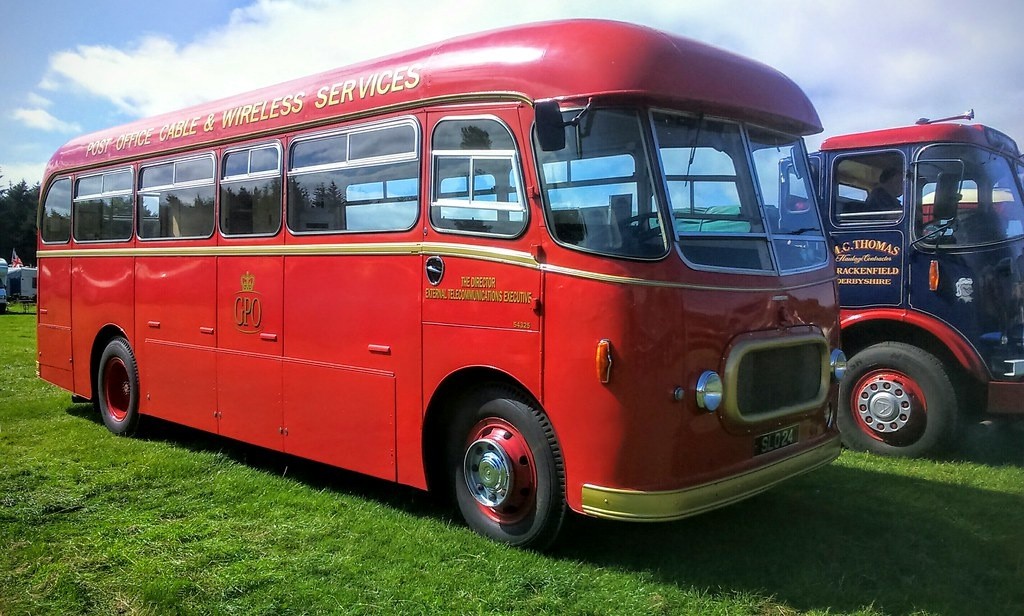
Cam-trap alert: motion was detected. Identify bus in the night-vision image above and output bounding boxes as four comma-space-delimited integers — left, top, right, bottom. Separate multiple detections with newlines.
35, 19, 848, 552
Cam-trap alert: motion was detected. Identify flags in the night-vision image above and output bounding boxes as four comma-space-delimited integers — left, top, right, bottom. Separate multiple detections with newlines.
11, 249, 24, 268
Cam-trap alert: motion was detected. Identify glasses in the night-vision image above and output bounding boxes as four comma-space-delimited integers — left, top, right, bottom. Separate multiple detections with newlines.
892, 180, 903, 185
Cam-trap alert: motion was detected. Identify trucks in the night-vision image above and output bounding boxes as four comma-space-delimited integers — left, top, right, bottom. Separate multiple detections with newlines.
552, 109, 1024, 458
0, 258, 8, 314
6, 267, 37, 303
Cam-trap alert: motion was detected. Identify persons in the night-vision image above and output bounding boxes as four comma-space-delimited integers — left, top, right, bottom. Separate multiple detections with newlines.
861, 167, 904, 220
